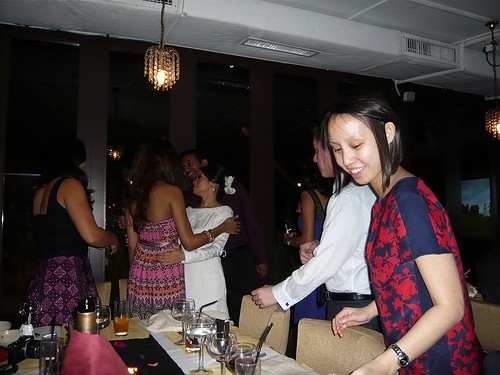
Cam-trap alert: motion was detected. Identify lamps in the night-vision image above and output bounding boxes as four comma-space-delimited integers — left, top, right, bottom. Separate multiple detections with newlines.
144, 0, 180, 91
486, 19, 500, 140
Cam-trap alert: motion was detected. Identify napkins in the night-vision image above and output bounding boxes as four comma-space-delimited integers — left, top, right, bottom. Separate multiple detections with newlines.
61, 330, 130, 375
144, 309, 182, 332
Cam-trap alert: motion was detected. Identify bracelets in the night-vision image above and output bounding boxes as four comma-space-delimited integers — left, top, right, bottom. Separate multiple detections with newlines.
203, 228, 216, 242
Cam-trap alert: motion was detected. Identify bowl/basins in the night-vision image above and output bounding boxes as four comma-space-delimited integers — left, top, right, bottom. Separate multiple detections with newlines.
0, 321, 11, 331
0, 329, 22, 348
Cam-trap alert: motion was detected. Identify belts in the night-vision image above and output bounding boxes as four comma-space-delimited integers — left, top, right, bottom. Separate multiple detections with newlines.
221, 246, 248, 258
323, 291, 372, 303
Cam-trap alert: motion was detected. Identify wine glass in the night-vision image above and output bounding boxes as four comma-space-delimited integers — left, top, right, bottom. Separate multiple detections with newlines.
94, 305, 109, 337
209, 332, 237, 375
186, 317, 214, 375
172, 298, 195, 345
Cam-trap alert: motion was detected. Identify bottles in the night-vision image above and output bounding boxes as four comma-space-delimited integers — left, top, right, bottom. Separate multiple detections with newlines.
76, 298, 96, 335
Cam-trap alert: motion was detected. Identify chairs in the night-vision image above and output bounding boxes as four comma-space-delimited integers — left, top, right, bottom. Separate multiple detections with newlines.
471, 300, 500, 352
94, 281, 111, 306
239, 295, 290, 356
119, 278, 128, 302
296, 318, 399, 375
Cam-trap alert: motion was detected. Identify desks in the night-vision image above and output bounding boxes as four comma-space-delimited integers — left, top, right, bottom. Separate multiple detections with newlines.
0, 318, 321, 375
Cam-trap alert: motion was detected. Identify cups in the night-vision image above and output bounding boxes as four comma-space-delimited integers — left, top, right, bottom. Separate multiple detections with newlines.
225, 342, 257, 374
234, 357, 261, 375
40, 338, 61, 375
113, 298, 130, 337
284, 221, 298, 245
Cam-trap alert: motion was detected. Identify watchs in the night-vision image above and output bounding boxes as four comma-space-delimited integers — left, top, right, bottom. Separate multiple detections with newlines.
287, 238, 291, 245
390, 344, 409, 367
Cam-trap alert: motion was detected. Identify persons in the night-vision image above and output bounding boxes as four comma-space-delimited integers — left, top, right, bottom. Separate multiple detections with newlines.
24, 136, 120, 328
157, 164, 234, 320
251, 126, 382, 335
318, 97, 485, 375
125, 139, 241, 320
118, 148, 269, 308
282, 152, 333, 324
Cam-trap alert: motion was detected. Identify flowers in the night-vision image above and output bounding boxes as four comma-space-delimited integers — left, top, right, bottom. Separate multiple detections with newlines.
224, 176, 236, 194
